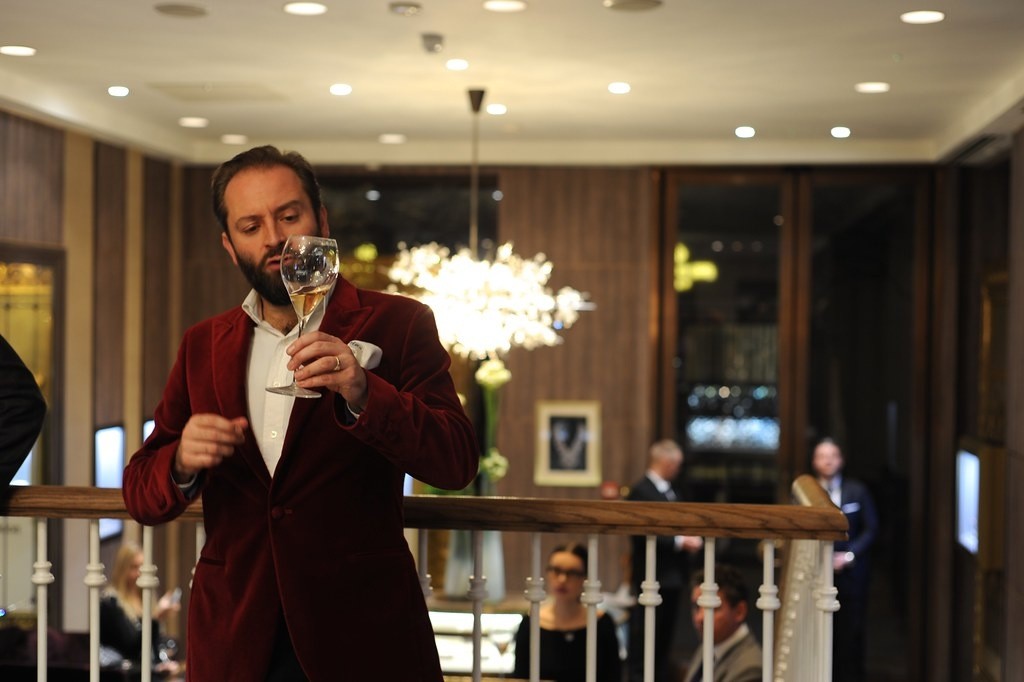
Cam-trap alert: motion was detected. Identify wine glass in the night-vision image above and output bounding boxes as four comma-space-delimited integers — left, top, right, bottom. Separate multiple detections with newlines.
265, 233, 340, 399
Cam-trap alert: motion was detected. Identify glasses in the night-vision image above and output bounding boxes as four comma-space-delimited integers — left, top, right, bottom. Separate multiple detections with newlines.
544, 565, 586, 583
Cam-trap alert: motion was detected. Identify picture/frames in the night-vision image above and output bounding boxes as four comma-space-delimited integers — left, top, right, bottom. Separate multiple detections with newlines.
533, 398, 601, 487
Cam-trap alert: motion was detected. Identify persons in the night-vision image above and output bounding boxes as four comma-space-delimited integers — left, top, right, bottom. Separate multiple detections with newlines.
0, 329, 48, 506
514, 437, 875, 682
90, 146, 477, 682
